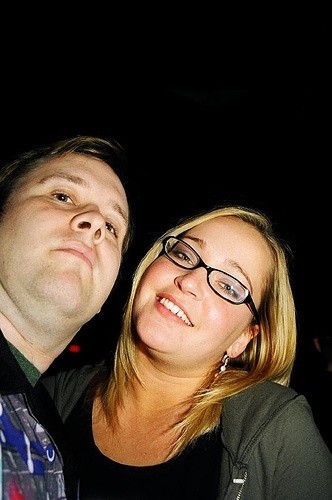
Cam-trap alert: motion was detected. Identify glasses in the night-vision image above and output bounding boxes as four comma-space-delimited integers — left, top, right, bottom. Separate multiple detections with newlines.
162, 236, 260, 325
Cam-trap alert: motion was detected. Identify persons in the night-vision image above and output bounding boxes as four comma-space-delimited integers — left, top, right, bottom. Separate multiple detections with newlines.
3, 133, 135, 500
44, 205, 332, 500
